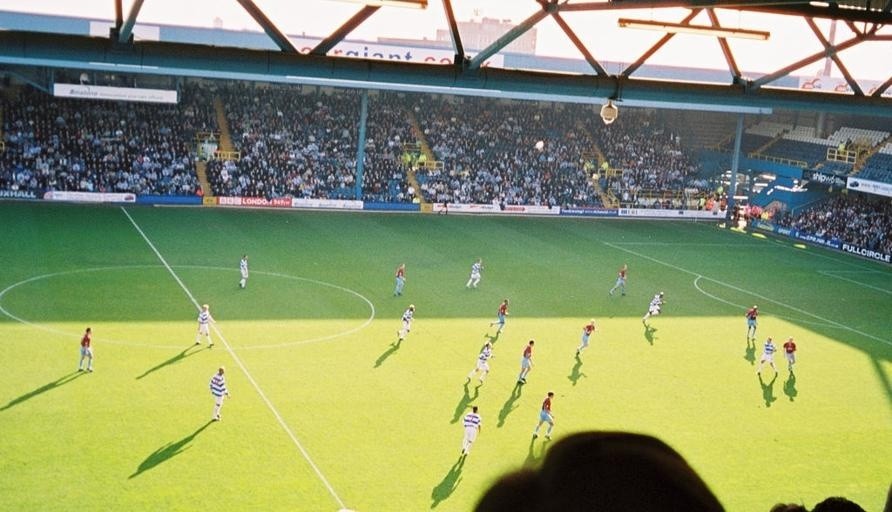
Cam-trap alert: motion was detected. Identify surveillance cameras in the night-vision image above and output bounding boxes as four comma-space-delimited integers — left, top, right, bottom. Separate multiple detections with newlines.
600, 101, 618, 124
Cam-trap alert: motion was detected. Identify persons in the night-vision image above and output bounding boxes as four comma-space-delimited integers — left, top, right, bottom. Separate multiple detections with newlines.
756, 335, 778, 375
642, 292, 665, 322
746, 305, 758, 340
465, 259, 482, 289
517, 339, 536, 383
783, 336, 796, 367
79, 328, 93, 372
490, 300, 509, 336
238, 255, 249, 287
732, 183, 891, 257
812, 498, 863, 510
1, 64, 726, 214
577, 320, 597, 353
467, 342, 494, 384
473, 432, 725, 511
209, 368, 228, 421
769, 502, 806, 511
609, 264, 627, 296
393, 264, 406, 296
397, 304, 416, 341
462, 407, 481, 457
533, 392, 555, 440
196, 305, 213, 348
